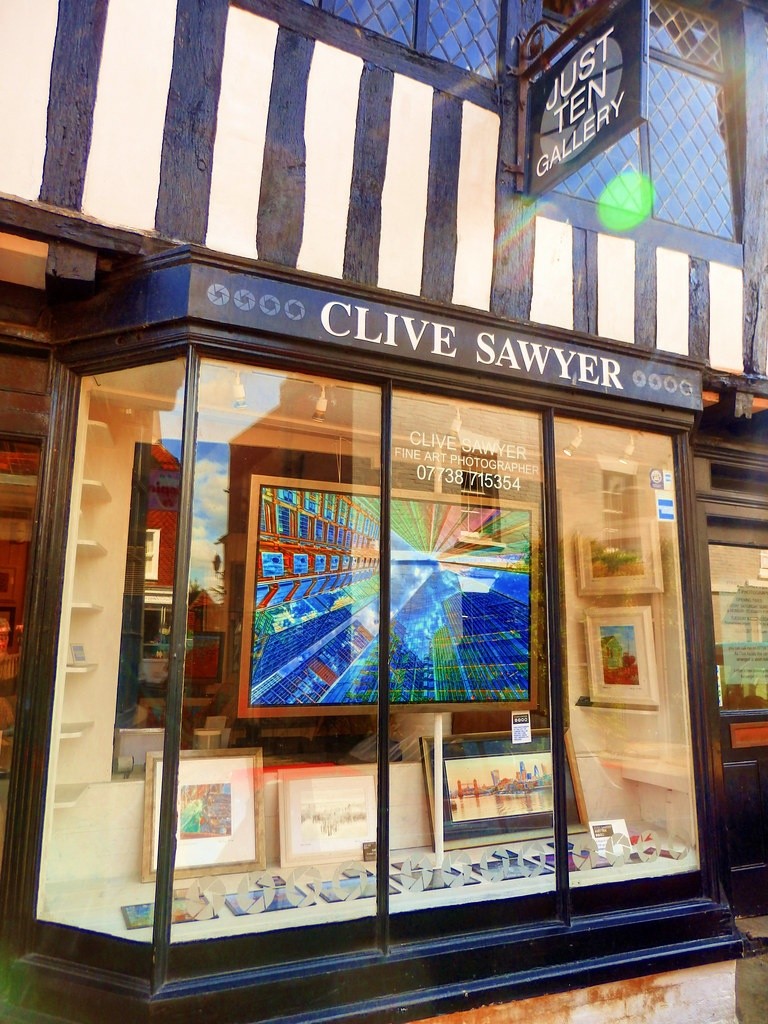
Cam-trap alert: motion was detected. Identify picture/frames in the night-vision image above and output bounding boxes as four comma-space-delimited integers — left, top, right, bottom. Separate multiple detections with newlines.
120, 474, 665, 930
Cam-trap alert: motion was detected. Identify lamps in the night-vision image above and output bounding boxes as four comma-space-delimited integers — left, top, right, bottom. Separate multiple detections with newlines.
233, 368, 635, 466
